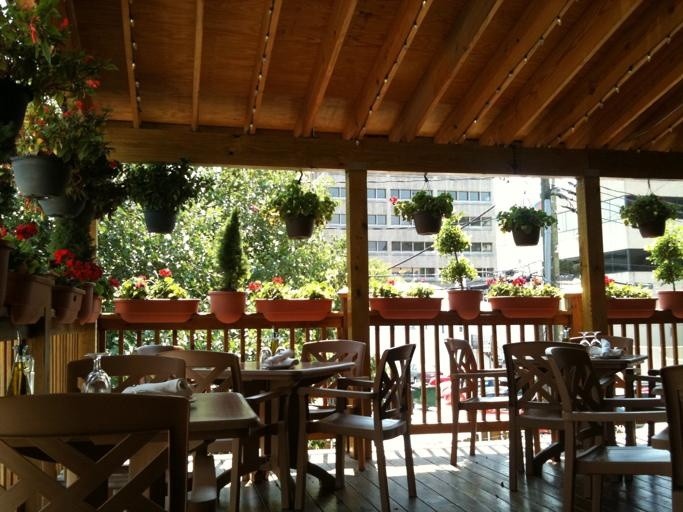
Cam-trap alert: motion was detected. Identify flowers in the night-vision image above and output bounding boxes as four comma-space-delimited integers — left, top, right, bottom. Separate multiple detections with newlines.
250, 276, 285, 293
488, 277, 526, 288
111, 268, 173, 295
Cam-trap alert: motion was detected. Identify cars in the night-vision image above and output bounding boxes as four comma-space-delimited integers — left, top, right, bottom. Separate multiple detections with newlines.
407, 359, 533, 421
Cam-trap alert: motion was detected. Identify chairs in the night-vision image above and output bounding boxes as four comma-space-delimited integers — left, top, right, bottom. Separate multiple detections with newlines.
441, 337, 681, 512
0, 385, 190, 511
66, 341, 418, 511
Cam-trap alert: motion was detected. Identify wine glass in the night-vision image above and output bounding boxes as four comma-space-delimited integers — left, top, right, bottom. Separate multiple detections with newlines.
82, 350, 110, 393
578, 331, 590, 346
590, 331, 600, 346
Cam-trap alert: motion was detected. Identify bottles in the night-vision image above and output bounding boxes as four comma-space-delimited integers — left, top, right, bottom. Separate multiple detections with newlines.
562, 326, 570, 343
4, 344, 31, 396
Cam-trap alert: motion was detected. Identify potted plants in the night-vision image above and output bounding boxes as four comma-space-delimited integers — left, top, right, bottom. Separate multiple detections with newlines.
3, 2, 126, 325
496, 205, 556, 246
620, 195, 681, 238
399, 191, 452, 235
272, 182, 335, 239
209, 208, 246, 324
124, 160, 208, 234
436, 221, 482, 320
649, 233, 683, 320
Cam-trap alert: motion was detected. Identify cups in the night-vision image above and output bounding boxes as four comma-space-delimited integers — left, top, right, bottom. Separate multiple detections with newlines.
587, 338, 622, 357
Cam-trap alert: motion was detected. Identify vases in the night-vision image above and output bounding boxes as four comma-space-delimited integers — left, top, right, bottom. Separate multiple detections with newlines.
490, 295, 561, 320
255, 299, 332, 325
114, 298, 199, 325
368, 298, 444, 321
602, 298, 656, 320
